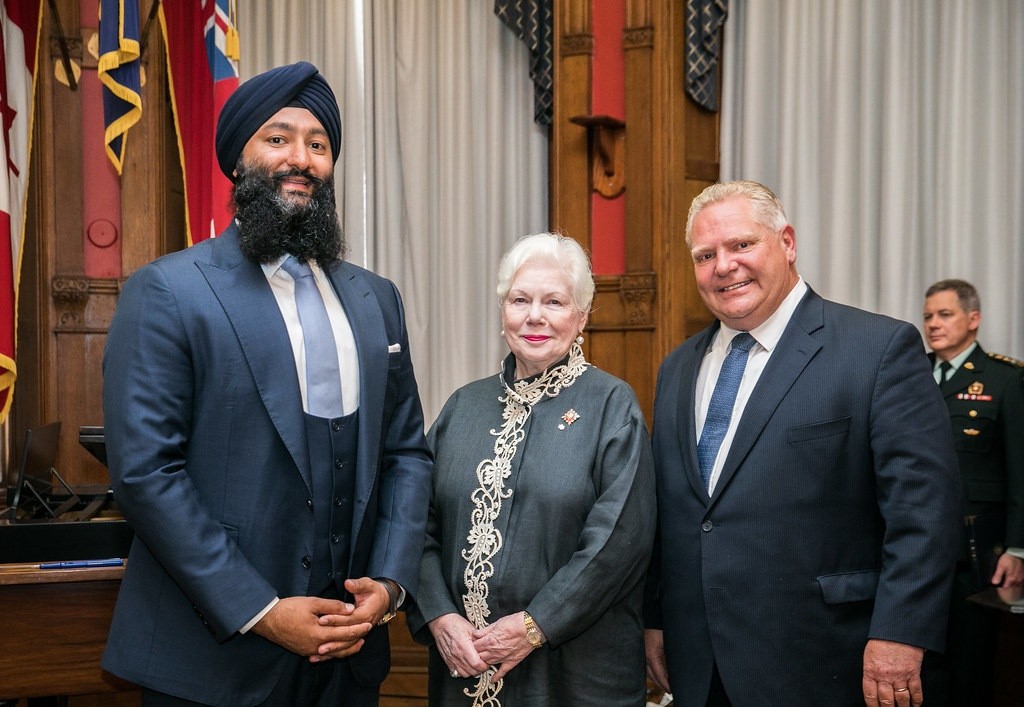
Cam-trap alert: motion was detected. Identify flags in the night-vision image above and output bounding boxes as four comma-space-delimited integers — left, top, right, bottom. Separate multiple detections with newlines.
158, 0, 241, 248
96, 0, 147, 176
0, 0, 43, 423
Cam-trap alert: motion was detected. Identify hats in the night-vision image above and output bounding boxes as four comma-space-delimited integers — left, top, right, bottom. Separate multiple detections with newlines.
216, 61, 342, 185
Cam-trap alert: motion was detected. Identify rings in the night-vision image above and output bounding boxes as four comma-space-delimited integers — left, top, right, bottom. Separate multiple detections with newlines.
451, 670, 458, 678
895, 688, 907, 692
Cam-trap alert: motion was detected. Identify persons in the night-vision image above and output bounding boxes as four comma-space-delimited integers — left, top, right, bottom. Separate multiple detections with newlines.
407, 231, 654, 707
641, 180, 961, 707
924, 277, 1024, 707
98, 60, 434, 707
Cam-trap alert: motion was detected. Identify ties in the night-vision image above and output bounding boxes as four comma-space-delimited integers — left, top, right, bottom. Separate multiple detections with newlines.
698, 334, 756, 492
938, 361, 952, 389
282, 257, 344, 418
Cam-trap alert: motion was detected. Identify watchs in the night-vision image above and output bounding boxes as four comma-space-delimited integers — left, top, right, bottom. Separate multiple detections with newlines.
523, 610, 542, 648
375, 578, 397, 625
